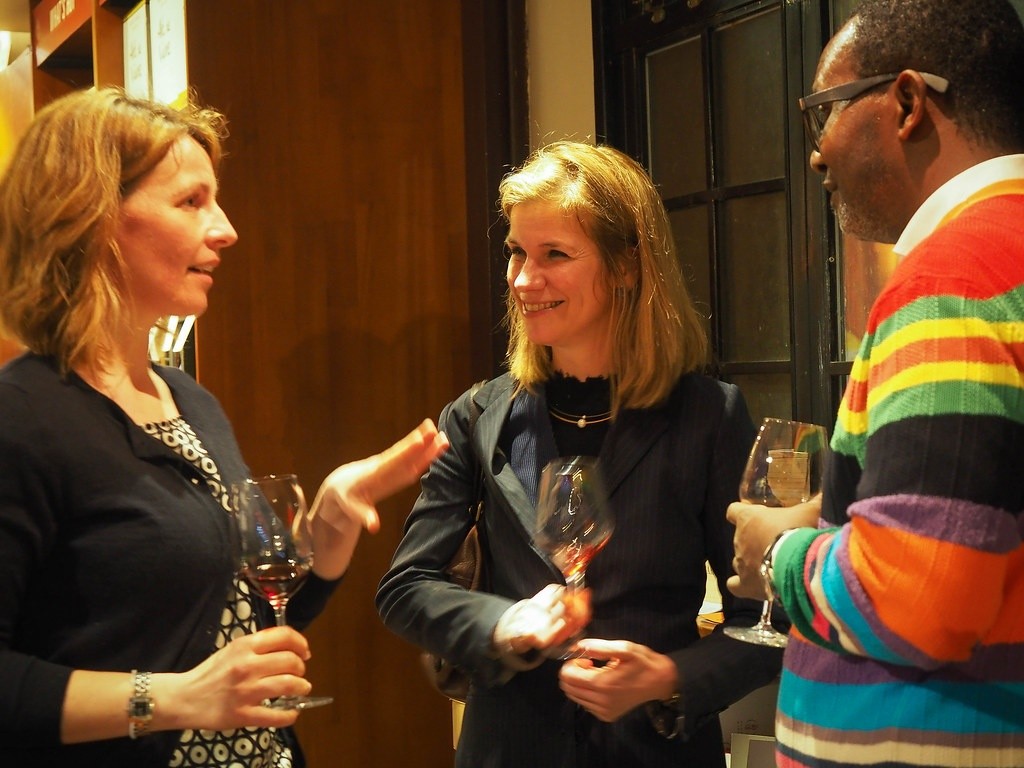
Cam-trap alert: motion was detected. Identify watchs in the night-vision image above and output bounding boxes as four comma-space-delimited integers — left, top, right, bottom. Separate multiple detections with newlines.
760, 523, 801, 603
130, 671, 156, 737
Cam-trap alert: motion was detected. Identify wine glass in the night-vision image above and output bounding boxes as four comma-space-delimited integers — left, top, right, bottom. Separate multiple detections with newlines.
531, 456, 612, 661
723, 417, 828, 652
229, 473, 336, 711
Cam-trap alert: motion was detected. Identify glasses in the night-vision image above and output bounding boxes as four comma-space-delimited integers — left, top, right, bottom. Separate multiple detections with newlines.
799, 69, 949, 153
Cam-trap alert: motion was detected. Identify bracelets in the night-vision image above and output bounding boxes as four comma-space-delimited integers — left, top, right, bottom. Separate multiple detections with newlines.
129, 670, 139, 738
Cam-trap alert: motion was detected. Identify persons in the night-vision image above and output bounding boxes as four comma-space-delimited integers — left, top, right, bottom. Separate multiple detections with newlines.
0, 84, 453, 767
723, 0, 1024, 768
373, 138, 793, 768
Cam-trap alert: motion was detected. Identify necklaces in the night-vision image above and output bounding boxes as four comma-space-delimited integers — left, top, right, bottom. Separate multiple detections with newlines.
546, 397, 615, 427
